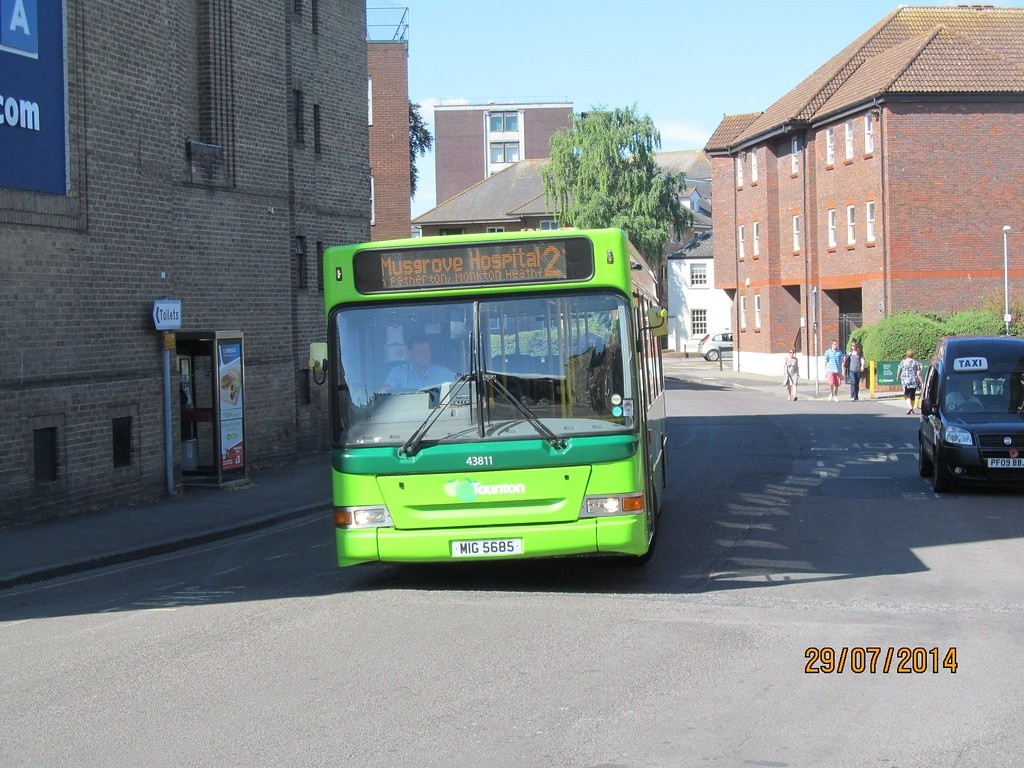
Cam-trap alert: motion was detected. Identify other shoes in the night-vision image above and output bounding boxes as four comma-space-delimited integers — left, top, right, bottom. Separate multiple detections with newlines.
911, 411, 915, 414
788, 397, 791, 400
850, 397, 854, 401
828, 395, 832, 401
794, 397, 798, 401
832, 396, 839, 402
907, 408, 913, 414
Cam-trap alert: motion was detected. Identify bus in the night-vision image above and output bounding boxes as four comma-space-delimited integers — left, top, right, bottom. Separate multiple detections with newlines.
309, 228, 670, 569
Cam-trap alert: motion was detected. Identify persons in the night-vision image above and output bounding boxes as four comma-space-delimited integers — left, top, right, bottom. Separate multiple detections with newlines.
783, 348, 800, 401
946, 375, 986, 411
896, 349, 924, 415
824, 339, 866, 403
383, 333, 458, 395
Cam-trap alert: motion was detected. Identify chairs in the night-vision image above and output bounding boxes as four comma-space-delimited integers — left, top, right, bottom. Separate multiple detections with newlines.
491, 354, 561, 407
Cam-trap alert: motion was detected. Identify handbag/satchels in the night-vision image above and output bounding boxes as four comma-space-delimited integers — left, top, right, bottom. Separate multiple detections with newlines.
917, 387, 924, 408
844, 353, 852, 367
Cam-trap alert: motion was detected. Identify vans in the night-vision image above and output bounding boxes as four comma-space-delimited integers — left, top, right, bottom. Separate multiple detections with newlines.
918, 335, 1024, 494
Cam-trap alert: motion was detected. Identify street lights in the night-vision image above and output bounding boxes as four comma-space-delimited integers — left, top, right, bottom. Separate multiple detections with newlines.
1002, 225, 1012, 336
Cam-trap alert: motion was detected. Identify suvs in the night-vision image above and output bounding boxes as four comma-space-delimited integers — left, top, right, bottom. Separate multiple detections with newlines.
698, 331, 733, 362
